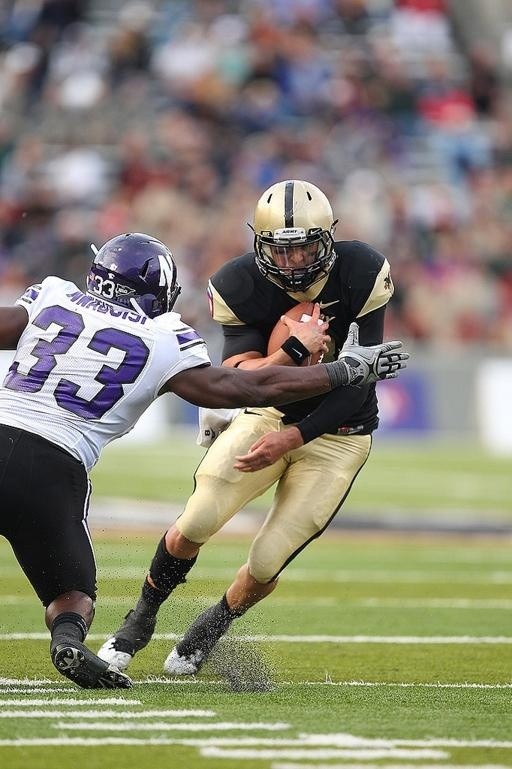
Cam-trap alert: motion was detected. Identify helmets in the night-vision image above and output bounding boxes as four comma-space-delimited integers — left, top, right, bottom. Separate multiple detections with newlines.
253, 177, 335, 269
85, 232, 177, 320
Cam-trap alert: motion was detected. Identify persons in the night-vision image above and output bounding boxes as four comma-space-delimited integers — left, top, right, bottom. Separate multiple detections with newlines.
95, 180, 394, 675
1, 234, 411, 691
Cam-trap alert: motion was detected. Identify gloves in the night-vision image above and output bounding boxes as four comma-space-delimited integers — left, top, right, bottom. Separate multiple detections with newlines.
337, 318, 409, 387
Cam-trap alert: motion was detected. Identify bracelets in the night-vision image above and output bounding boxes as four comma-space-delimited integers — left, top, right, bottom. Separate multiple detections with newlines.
280, 336, 310, 366
323, 361, 348, 389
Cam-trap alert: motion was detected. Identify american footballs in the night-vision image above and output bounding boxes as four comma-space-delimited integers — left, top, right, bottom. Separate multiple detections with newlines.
265, 301, 328, 368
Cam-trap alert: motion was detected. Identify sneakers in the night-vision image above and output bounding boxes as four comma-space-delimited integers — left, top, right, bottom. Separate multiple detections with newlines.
49, 636, 134, 690
96, 607, 157, 671
162, 607, 229, 677
